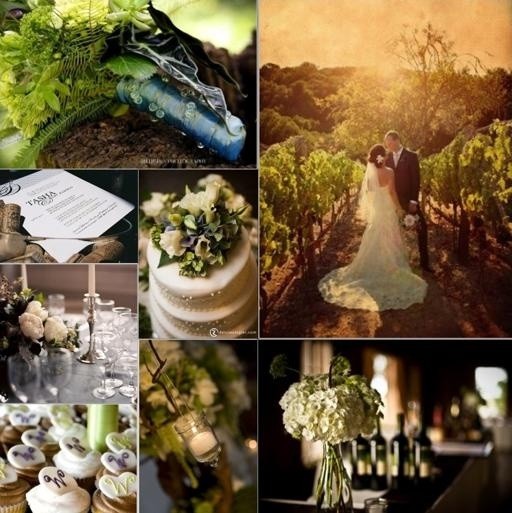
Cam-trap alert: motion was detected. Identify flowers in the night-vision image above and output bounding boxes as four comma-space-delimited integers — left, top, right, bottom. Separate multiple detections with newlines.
266, 350, 398, 504
0, 286, 84, 360
149, 185, 252, 280
399, 213, 421, 231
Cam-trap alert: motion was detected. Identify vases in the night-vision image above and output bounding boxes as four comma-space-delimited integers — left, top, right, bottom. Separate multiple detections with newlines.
311, 452, 355, 507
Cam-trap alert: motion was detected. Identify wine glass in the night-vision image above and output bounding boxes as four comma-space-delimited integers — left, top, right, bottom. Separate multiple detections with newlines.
8, 293, 138, 403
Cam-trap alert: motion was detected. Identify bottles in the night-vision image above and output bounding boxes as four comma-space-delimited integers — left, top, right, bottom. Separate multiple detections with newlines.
350, 412, 433, 491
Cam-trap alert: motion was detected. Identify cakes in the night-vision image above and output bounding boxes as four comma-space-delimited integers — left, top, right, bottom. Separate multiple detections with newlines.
145, 225, 258, 340
0, 406, 137, 513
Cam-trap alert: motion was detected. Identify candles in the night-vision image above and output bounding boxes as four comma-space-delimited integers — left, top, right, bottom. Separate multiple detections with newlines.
87, 264, 95, 295
20, 265, 28, 291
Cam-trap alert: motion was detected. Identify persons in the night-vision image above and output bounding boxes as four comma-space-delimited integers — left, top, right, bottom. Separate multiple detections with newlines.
383, 128, 434, 274
317, 144, 429, 311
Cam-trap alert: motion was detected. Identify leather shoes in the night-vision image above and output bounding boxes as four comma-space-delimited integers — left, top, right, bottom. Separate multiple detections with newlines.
422, 263, 435, 273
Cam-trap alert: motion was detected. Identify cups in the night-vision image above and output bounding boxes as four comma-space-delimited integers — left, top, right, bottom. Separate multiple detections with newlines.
174, 409, 224, 464
